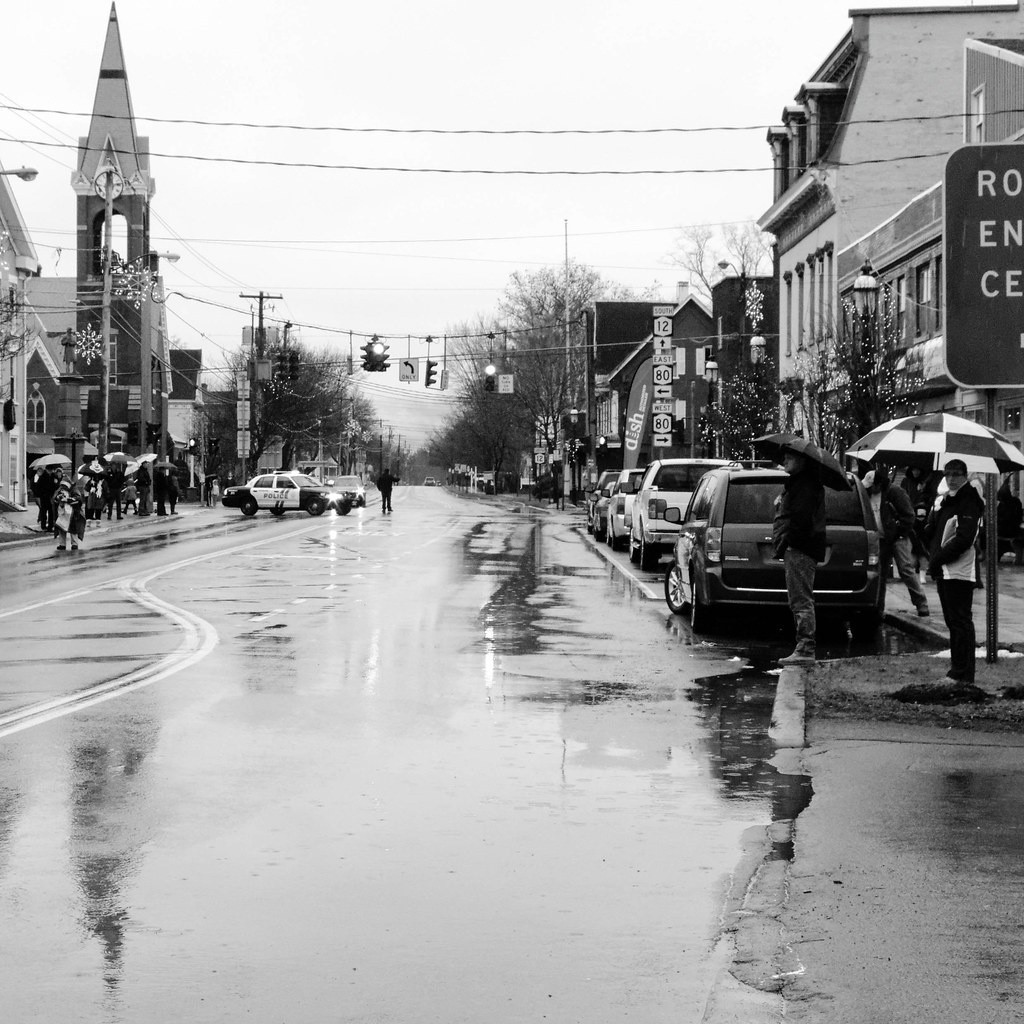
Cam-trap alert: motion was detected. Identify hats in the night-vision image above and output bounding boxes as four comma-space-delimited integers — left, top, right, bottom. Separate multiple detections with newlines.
862, 469, 886, 489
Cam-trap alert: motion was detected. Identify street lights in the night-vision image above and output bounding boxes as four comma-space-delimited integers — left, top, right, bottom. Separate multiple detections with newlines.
748, 329, 769, 436
99, 250, 182, 455
571, 406, 579, 508
853, 260, 880, 434
718, 261, 744, 366
705, 355, 718, 458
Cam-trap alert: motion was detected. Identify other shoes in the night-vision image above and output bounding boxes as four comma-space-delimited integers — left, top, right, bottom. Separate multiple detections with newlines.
57, 545, 66, 550
41, 528, 53, 531
917, 608, 930, 617
157, 513, 169, 516
777, 652, 816, 667
107, 516, 123, 519
171, 512, 178, 515
139, 512, 150, 516
71, 545, 78, 549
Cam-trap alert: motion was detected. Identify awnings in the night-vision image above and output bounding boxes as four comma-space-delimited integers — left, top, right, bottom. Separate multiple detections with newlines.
817, 335, 959, 415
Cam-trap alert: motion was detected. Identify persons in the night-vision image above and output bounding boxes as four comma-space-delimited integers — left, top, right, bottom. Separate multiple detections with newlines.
376, 469, 400, 513
34, 468, 233, 551
862, 460, 985, 682
776, 449, 827, 662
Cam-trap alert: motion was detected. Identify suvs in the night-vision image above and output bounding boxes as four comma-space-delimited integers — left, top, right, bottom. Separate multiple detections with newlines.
663, 461, 889, 637
621, 458, 746, 569
601, 468, 649, 549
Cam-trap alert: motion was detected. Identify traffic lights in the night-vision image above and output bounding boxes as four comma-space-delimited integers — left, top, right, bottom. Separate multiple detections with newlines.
189, 439, 198, 454
209, 437, 218, 453
361, 342, 372, 370
371, 344, 383, 370
274, 353, 287, 378
148, 421, 162, 443
425, 361, 437, 387
484, 365, 496, 391
383, 346, 390, 368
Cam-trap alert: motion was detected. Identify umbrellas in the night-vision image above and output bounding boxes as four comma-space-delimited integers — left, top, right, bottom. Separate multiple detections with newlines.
751, 432, 855, 493
845, 412, 1024, 518
29, 452, 178, 476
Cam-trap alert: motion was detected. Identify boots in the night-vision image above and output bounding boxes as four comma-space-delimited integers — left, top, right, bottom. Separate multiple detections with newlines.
96, 520, 101, 527
122, 509, 127, 514
86, 519, 91, 528
133, 509, 137, 515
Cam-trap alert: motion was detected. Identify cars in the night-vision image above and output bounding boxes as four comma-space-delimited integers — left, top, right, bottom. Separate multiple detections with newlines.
330, 476, 368, 506
222, 469, 350, 516
585, 471, 621, 541
424, 477, 436, 485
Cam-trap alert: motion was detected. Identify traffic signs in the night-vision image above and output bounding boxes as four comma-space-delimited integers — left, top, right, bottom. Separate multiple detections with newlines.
399, 358, 418, 381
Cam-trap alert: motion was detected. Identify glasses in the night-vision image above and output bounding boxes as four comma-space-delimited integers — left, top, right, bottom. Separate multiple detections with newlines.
944, 472, 964, 477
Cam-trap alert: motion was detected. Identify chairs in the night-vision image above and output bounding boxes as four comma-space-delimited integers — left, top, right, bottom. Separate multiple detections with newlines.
661, 473, 676, 489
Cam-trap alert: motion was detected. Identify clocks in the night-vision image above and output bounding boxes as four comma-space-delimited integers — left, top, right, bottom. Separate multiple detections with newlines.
94, 171, 123, 201
